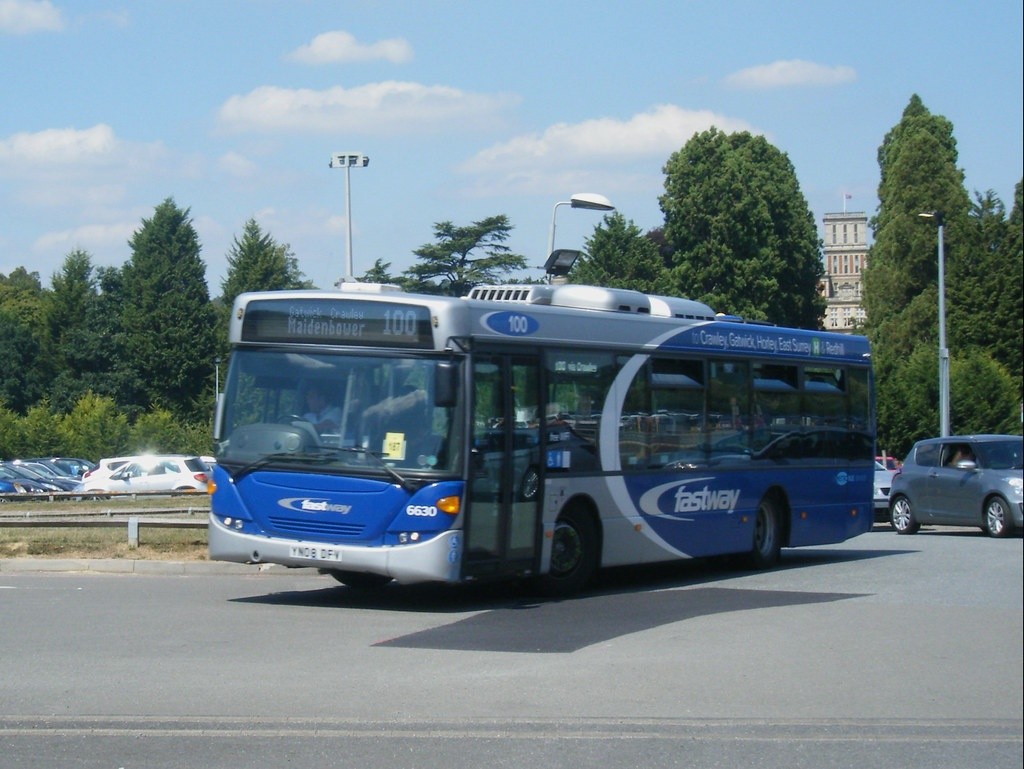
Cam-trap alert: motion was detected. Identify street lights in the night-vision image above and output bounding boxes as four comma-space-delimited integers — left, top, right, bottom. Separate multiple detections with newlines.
547, 193, 616, 286
329, 150, 370, 279
915, 208, 951, 437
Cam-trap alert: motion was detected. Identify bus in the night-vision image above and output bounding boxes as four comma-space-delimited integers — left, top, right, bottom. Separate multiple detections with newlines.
208, 282, 880, 606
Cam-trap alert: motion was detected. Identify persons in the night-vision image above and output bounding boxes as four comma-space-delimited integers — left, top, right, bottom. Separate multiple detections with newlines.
303, 386, 342, 435
952, 450, 963, 467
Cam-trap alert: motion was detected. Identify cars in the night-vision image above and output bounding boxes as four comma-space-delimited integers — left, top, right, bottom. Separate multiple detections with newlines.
0, 461, 74, 502
888, 434, 1024, 539
69, 455, 212, 502
873, 461, 895, 523
875, 456, 899, 475
0, 455, 217, 492
